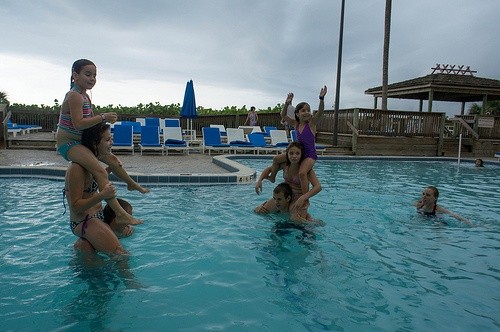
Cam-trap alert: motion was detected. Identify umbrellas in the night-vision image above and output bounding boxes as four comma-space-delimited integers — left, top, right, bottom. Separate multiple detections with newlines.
180, 79, 196, 142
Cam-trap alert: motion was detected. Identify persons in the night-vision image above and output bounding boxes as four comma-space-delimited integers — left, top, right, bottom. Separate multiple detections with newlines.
418, 186, 464, 222
54, 123, 150, 260
55, 59, 149, 225
473, 159, 485, 167
244, 106, 258, 126
253, 144, 322, 224
264, 86, 327, 210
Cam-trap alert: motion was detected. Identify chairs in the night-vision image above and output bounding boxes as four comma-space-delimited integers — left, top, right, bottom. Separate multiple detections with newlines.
7, 120, 42, 136
110, 116, 327, 157
